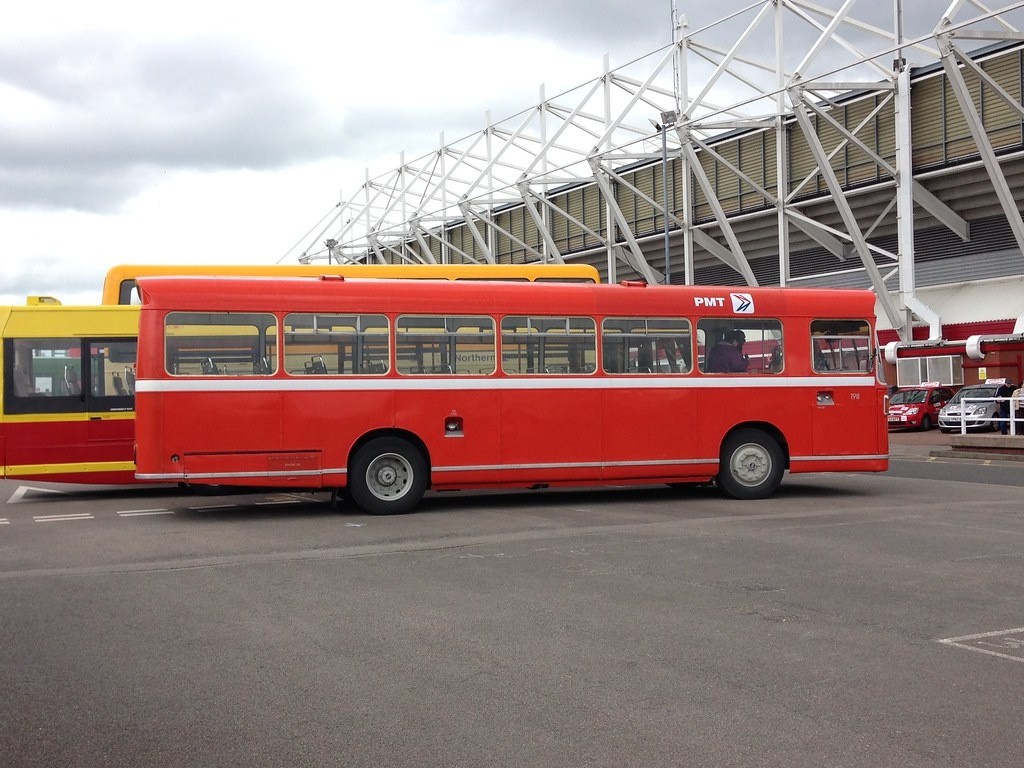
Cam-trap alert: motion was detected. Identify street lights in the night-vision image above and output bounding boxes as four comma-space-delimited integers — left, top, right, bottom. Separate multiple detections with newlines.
648, 110, 678, 285
327, 239, 338, 265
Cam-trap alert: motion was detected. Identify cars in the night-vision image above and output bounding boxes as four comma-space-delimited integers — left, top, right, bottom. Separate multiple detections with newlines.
938, 378, 1015, 433
887, 381, 958, 431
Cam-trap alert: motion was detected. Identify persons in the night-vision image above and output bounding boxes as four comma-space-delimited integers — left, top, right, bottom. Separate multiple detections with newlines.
994, 379, 1024, 435
707, 331, 750, 373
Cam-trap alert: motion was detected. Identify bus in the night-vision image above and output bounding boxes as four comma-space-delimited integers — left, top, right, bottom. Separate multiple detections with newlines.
134, 274, 890, 515
100, 264, 601, 395
0, 296, 702, 497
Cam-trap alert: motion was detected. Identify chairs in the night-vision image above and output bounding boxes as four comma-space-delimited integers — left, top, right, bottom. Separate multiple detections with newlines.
165, 341, 832, 378
65, 365, 80, 395
112, 372, 128, 396
125, 366, 134, 396
